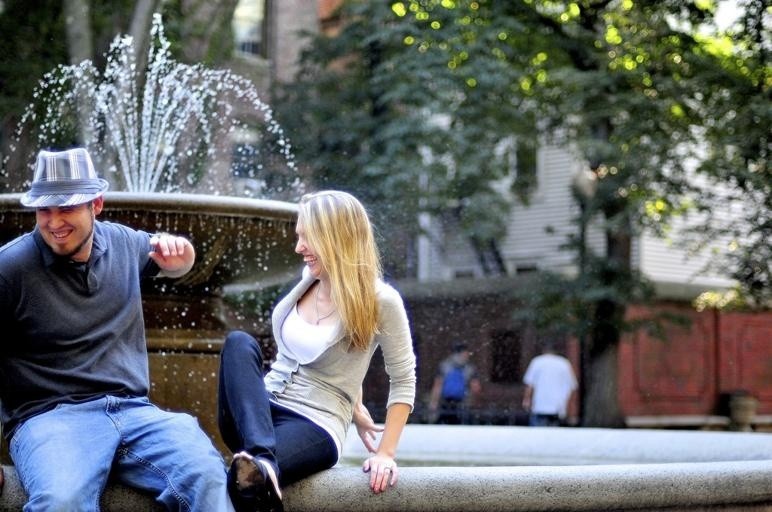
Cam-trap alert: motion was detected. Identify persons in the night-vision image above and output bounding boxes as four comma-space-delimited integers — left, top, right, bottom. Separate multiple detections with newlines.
212, 186, 418, 511
518, 337, 579, 426
1, 148, 236, 512
433, 342, 483, 424
485, 330, 520, 426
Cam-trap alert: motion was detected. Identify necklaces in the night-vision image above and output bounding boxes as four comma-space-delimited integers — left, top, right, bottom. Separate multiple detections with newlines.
312, 281, 337, 326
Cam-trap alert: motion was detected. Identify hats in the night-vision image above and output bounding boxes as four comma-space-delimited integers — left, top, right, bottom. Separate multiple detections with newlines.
19, 147, 109, 209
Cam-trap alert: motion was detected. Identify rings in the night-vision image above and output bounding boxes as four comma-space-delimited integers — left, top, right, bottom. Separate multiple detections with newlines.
384, 466, 391, 470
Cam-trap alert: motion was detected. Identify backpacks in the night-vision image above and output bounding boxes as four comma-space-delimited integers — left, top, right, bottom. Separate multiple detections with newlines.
441, 363, 466, 403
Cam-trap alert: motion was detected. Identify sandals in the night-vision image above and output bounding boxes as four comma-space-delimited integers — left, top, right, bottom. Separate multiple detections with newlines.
226, 453, 283, 512
